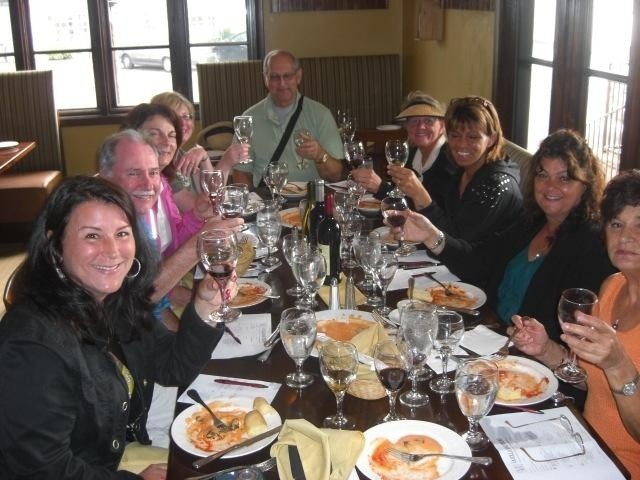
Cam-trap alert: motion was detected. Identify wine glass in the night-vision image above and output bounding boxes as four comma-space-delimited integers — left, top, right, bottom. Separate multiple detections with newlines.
293, 128, 312, 174
352, 229, 381, 292
264, 164, 282, 210
197, 228, 242, 321
233, 115, 255, 164
271, 161, 288, 204
373, 304, 503, 456
277, 305, 319, 390
201, 169, 224, 216
381, 197, 416, 256
371, 251, 398, 318
295, 254, 328, 310
291, 246, 320, 304
255, 207, 282, 266
317, 342, 361, 433
282, 234, 308, 297
552, 284, 602, 384
363, 238, 389, 308
385, 139, 409, 198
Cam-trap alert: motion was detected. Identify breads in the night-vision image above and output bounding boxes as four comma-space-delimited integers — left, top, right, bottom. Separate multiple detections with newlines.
495, 386, 520, 400
406, 287, 435, 303
254, 397, 277, 416
246, 411, 266, 436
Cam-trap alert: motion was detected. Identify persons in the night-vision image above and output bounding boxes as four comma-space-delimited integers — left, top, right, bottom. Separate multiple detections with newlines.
383, 128, 623, 353
151, 90, 252, 214
93, 130, 246, 451
1, 174, 241, 479
351, 91, 448, 212
384, 95, 527, 243
231, 48, 345, 189
507, 168, 640, 480
108, 102, 217, 321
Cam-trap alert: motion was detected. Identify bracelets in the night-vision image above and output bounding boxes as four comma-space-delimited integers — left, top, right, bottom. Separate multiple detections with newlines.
200, 155, 210, 162
428, 229, 446, 254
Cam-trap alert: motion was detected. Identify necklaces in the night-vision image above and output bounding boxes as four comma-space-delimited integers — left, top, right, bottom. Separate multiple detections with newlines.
534, 228, 552, 260
610, 299, 635, 333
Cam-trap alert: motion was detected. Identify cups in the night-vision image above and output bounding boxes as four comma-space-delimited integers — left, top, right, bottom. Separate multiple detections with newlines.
216, 186, 245, 220
337, 109, 358, 142
230, 182, 249, 208
344, 140, 365, 169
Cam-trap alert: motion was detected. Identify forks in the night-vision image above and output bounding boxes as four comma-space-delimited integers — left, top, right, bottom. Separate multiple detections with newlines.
424, 271, 454, 297
384, 445, 494, 468
187, 456, 277, 479
484, 316, 531, 362
182, 385, 228, 431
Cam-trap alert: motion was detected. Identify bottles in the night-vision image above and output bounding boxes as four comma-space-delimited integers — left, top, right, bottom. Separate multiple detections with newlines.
317, 193, 343, 285
339, 185, 367, 268
300, 180, 316, 238
310, 179, 326, 252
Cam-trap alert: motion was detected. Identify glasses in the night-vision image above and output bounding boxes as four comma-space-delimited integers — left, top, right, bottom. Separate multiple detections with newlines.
450, 97, 489, 109
265, 72, 297, 82
181, 113, 193, 121
505, 415, 586, 463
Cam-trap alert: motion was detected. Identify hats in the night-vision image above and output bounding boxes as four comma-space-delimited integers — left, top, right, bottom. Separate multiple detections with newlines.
393, 96, 446, 121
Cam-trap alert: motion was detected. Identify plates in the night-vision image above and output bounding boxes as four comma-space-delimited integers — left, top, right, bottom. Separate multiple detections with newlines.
169, 395, 285, 459
354, 418, 473, 480
302, 309, 376, 360
350, 197, 383, 214
278, 180, 309, 198
325, 179, 366, 195
420, 280, 488, 311
372, 226, 419, 249
240, 192, 266, 217
472, 354, 560, 406
227, 277, 272, 309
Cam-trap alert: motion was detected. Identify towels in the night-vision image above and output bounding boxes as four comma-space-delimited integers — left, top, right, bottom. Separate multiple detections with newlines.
318, 271, 368, 308
324, 322, 410, 382
115, 441, 168, 476
270, 418, 367, 480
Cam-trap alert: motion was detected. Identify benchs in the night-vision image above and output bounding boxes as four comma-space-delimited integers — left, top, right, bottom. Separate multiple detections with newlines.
0, 69, 66, 232
196, 48, 405, 164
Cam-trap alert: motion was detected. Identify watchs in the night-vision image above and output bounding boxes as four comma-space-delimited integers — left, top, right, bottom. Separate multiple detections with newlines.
610, 373, 638, 399
316, 152, 329, 165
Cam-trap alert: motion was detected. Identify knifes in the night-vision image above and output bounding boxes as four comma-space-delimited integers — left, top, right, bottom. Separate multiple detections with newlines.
191, 423, 286, 473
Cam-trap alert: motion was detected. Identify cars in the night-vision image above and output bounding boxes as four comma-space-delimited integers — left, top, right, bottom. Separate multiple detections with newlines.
212, 31, 249, 62
118, 49, 171, 72
